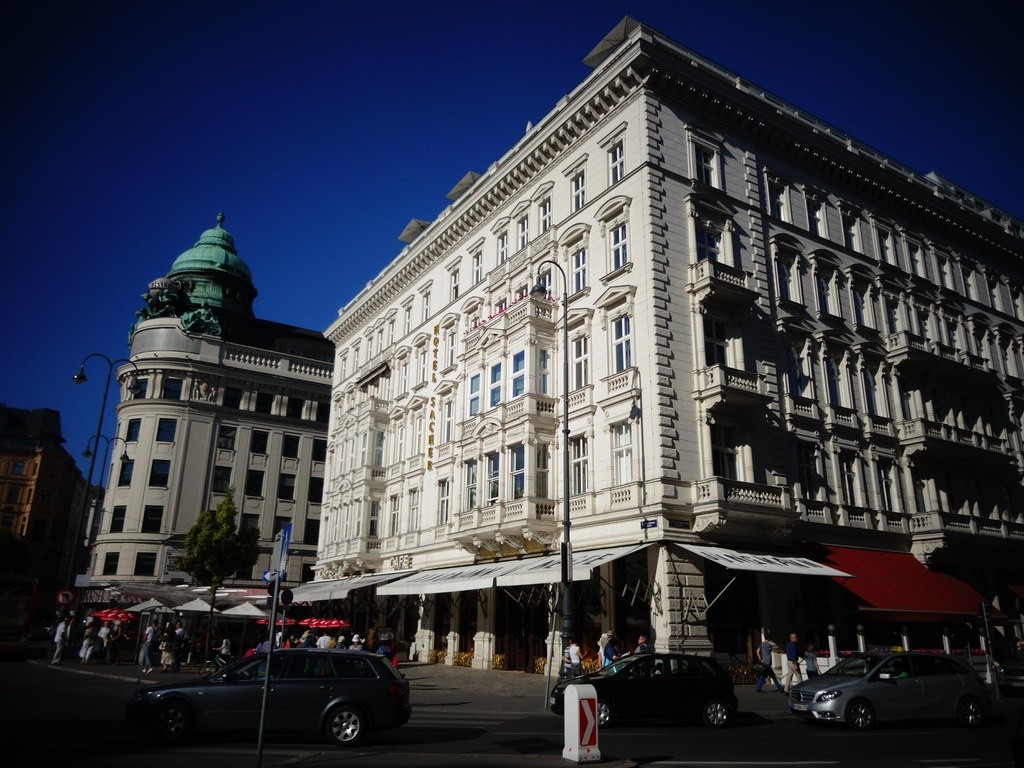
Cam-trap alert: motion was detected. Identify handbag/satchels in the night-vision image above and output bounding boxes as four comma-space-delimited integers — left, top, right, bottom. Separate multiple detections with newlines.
159, 633, 169, 651
754, 663, 768, 675
376, 646, 391, 656
392, 656, 398, 667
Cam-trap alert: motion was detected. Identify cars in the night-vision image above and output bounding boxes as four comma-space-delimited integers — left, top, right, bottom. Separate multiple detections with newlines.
1000, 649, 1024, 696
786, 650, 992, 733
550, 651, 739, 728
129, 647, 412, 750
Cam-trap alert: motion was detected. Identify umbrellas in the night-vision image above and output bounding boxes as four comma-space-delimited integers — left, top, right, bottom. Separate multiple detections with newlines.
89, 597, 349, 643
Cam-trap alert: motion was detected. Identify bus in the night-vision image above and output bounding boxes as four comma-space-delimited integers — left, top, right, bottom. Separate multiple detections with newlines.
0, 574, 41, 662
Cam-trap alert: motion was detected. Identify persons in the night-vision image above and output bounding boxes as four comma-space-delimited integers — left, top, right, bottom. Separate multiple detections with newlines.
785, 633, 802, 696
138, 619, 207, 674
562, 638, 588, 678
756, 632, 784, 692
634, 634, 649, 654
596, 631, 622, 671
216, 639, 234, 672
804, 645, 818, 679
243, 630, 363, 659
50, 615, 121, 666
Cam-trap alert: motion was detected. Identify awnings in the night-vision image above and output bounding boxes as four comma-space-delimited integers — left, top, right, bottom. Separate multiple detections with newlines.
1008, 584, 1023, 596
279, 570, 418, 606
812, 544, 1008, 622
674, 541, 854, 577
377, 543, 655, 596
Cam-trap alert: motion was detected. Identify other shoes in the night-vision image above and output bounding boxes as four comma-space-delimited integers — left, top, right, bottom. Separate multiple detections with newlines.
142, 668, 153, 673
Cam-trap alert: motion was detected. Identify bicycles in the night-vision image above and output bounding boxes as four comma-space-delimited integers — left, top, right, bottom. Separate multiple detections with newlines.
200, 647, 232, 679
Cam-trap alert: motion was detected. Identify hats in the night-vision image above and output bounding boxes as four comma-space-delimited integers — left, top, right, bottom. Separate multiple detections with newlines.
338, 636, 345, 642
352, 634, 360, 642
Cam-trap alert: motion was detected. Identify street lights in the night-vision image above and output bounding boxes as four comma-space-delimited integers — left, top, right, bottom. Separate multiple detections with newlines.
65, 353, 145, 585
81, 434, 130, 553
530, 260, 571, 682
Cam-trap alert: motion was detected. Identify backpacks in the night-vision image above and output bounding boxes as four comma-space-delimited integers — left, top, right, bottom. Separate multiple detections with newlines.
149, 626, 159, 645
